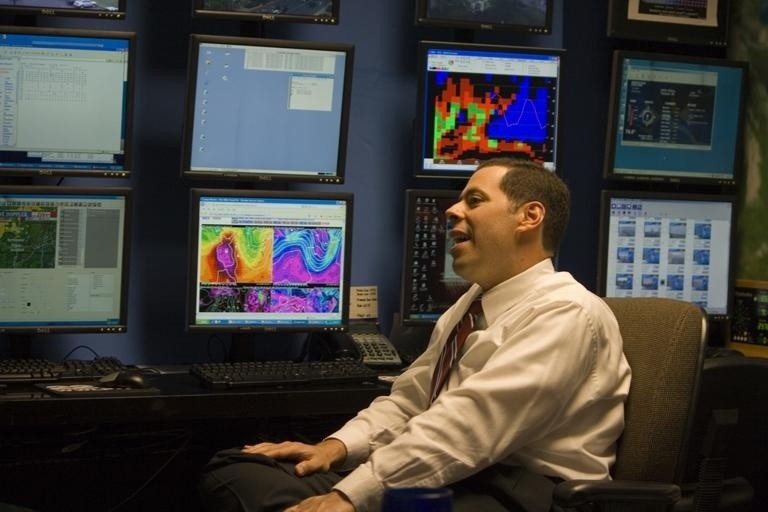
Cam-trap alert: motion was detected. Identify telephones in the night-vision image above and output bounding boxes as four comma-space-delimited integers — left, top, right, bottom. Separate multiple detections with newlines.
325, 321, 403, 368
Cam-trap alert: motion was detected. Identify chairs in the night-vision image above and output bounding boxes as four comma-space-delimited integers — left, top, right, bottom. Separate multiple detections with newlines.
515, 296, 708, 510
683, 355, 767, 512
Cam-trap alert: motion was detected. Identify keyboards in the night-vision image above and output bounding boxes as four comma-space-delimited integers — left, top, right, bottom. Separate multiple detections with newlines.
189, 360, 379, 387
1, 358, 124, 383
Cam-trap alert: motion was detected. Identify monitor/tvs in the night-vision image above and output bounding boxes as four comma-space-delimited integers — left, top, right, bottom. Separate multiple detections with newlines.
191, 0, 340, 27
599, 187, 737, 321
603, 46, 750, 188
0, 0, 130, 21
607, 0, 732, 54
181, 32, 355, 183
412, 40, 568, 183
188, 187, 354, 334
415, 0, 555, 37
0, 25, 136, 178
0, 183, 136, 335
401, 188, 472, 327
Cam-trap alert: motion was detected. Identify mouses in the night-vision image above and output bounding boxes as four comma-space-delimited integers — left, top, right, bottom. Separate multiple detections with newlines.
123, 373, 148, 388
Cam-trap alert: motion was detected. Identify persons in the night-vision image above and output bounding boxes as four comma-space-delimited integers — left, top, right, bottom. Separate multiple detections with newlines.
199, 158, 635, 511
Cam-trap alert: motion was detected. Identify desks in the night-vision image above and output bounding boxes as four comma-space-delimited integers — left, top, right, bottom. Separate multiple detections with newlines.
1, 364, 387, 425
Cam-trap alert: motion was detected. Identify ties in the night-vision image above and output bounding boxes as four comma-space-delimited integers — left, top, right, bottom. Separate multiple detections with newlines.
428, 291, 482, 409
